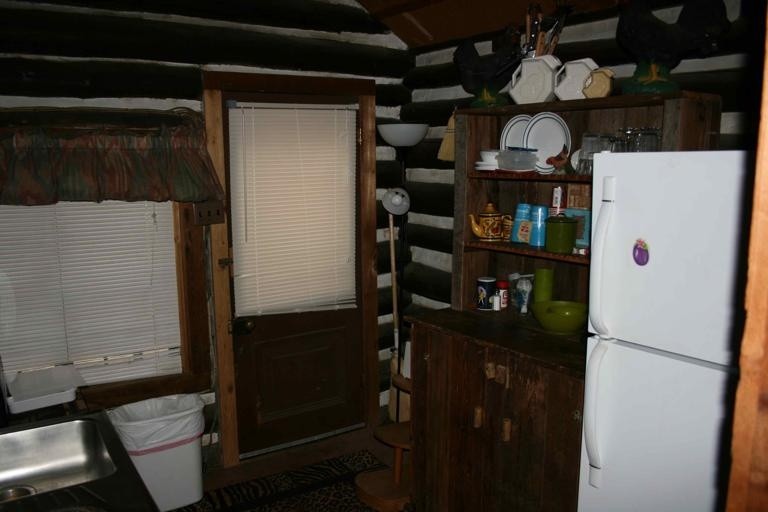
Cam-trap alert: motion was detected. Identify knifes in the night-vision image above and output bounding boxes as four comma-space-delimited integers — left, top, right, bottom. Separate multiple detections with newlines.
527, 17, 540, 58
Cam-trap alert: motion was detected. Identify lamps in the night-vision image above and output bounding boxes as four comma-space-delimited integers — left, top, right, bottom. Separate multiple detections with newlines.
376, 121, 430, 217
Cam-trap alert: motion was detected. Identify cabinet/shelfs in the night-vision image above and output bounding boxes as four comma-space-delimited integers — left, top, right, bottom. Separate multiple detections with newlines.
450, 90, 720, 343
403, 307, 585, 512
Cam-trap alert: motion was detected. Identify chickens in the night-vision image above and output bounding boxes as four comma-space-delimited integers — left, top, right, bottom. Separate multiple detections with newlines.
546, 144, 569, 169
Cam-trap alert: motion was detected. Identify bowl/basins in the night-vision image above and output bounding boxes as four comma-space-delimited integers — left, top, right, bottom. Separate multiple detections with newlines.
527, 299, 587, 337
479, 149, 501, 163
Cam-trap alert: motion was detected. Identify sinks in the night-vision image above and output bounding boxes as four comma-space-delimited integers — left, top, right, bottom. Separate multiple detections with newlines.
0, 410, 131, 505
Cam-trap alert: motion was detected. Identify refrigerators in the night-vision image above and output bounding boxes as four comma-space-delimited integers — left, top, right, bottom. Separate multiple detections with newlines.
574, 147, 750, 512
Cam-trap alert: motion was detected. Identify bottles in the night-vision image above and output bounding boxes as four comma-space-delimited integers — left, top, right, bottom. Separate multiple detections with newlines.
507, 272, 521, 306
476, 277, 496, 311
495, 280, 508, 309
515, 277, 533, 315
520, 274, 535, 283
511, 203, 531, 244
491, 288, 502, 312
529, 205, 547, 247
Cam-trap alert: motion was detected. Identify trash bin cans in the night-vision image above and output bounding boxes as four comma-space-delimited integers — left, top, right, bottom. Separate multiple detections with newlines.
107, 392, 207, 512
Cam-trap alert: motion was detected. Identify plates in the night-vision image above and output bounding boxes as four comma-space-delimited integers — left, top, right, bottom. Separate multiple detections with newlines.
497, 168, 537, 173
474, 161, 499, 171
499, 113, 532, 153
523, 111, 572, 175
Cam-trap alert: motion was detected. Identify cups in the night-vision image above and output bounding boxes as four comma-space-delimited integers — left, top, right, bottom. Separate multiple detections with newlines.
570, 126, 661, 178
543, 212, 577, 255
533, 268, 555, 303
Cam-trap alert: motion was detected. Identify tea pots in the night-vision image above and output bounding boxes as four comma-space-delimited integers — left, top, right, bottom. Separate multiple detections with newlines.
467, 203, 512, 242
